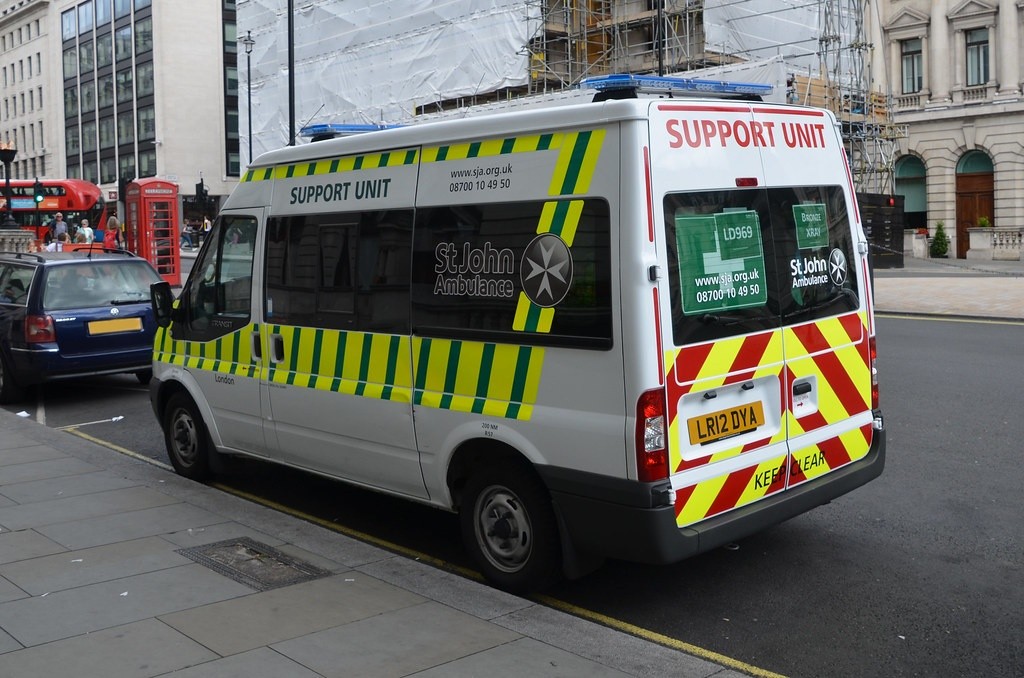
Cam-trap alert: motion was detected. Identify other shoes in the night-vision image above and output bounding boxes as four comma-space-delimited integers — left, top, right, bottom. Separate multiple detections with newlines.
192, 248, 197, 252
180, 248, 183, 252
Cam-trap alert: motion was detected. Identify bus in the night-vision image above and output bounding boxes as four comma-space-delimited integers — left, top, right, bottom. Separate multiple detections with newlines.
0, 178, 108, 241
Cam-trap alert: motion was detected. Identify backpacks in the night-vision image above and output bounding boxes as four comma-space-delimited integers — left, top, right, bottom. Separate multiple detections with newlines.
104, 228, 119, 253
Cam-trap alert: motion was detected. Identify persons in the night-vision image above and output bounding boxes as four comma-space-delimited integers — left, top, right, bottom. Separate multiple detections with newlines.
103, 216, 123, 254
180, 219, 197, 253
225, 226, 243, 248
199, 215, 212, 243
74, 219, 95, 244
46, 233, 90, 253
52, 212, 68, 239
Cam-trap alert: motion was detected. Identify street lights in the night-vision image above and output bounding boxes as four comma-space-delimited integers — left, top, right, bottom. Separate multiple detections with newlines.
243, 30, 255, 165
0, 148, 21, 230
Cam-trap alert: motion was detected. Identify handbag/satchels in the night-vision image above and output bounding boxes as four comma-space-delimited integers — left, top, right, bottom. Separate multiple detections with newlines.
77, 232, 85, 243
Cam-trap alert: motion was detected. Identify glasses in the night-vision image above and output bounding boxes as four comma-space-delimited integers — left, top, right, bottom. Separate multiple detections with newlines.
56, 216, 63, 218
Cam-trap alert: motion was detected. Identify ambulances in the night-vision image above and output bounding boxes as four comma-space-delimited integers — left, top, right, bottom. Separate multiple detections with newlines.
148, 74, 887, 595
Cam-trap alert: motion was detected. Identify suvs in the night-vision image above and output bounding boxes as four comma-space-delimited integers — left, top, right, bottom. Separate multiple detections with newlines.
0, 246, 180, 402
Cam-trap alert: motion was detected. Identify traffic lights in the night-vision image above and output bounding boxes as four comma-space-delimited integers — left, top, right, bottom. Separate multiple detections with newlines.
34, 182, 44, 203
